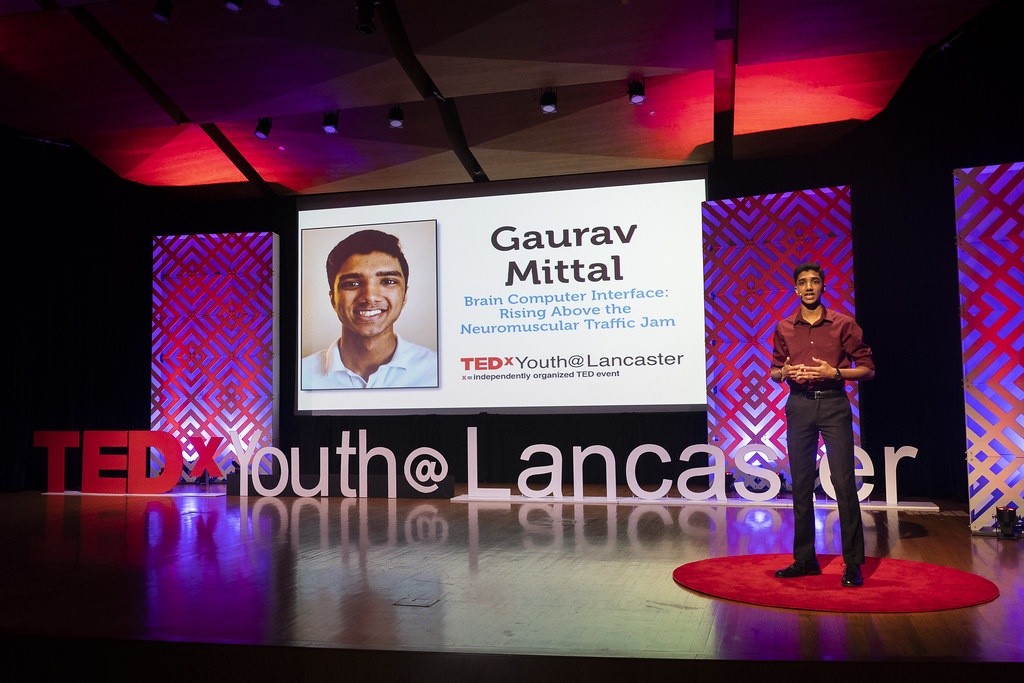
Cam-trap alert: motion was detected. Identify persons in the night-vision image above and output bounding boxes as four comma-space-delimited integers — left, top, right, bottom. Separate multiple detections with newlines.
303, 230, 438, 387
769, 264, 875, 586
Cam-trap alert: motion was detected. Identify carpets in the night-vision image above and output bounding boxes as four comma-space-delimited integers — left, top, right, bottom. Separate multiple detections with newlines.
672, 554, 1000, 614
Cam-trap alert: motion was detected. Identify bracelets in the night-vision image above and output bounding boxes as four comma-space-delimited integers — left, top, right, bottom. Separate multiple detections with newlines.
780, 368, 782, 377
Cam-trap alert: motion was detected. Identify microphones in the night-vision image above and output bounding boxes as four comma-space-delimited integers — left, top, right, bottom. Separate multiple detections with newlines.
796, 292, 802, 296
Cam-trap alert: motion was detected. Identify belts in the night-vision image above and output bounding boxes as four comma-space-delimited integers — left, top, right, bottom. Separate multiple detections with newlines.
790, 388, 844, 399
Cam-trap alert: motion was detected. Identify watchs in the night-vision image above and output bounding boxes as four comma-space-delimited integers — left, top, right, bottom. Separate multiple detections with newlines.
835, 369, 841, 380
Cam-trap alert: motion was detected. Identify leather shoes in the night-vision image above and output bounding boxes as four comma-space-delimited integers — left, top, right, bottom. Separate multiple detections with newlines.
841, 562, 864, 587
775, 559, 821, 578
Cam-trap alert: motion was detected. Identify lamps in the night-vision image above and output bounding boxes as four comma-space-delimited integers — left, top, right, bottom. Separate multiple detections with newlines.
993, 506, 1021, 539
538, 88, 558, 115
627, 78, 646, 105
385, 103, 404, 129
320, 111, 339, 135
255, 117, 272, 139
